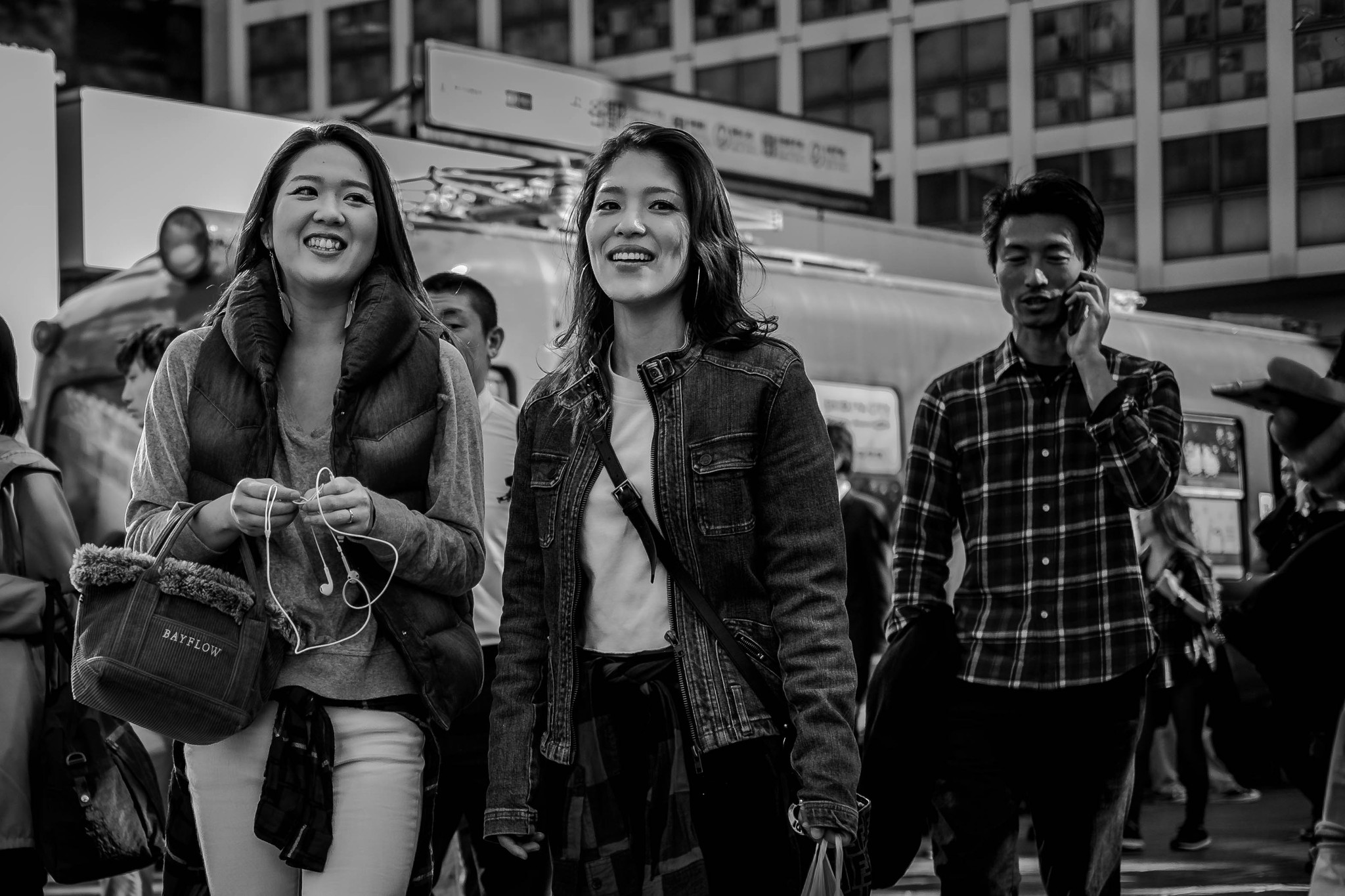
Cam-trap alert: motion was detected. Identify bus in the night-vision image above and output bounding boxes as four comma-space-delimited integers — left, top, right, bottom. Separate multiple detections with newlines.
18, 150, 1339, 611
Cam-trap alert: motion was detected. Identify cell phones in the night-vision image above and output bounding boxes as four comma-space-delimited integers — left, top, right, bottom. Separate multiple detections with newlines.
1068, 267, 1093, 335
1211, 379, 1345, 420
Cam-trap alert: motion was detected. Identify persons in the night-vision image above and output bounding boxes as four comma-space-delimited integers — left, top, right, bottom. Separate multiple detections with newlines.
118, 321, 184, 429
1205, 346, 1344, 896
824, 420, 966, 729
0, 320, 88, 896
490, 117, 870, 895
1119, 490, 1222, 853
402, 269, 522, 896
862, 168, 1183, 894
127, 121, 487, 895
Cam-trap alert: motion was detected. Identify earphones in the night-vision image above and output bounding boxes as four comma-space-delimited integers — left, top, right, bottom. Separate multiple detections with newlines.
341, 557, 359, 584
319, 567, 334, 596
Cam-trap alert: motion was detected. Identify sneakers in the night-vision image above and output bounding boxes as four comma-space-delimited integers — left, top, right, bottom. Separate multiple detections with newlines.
1207, 787, 1263, 806
1152, 781, 1189, 806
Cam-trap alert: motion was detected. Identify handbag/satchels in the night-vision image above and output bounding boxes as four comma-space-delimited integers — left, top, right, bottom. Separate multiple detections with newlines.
28, 577, 170, 887
68, 497, 312, 748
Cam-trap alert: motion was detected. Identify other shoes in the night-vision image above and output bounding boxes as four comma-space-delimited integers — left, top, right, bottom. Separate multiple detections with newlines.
1170, 824, 1212, 852
1121, 826, 1147, 853
